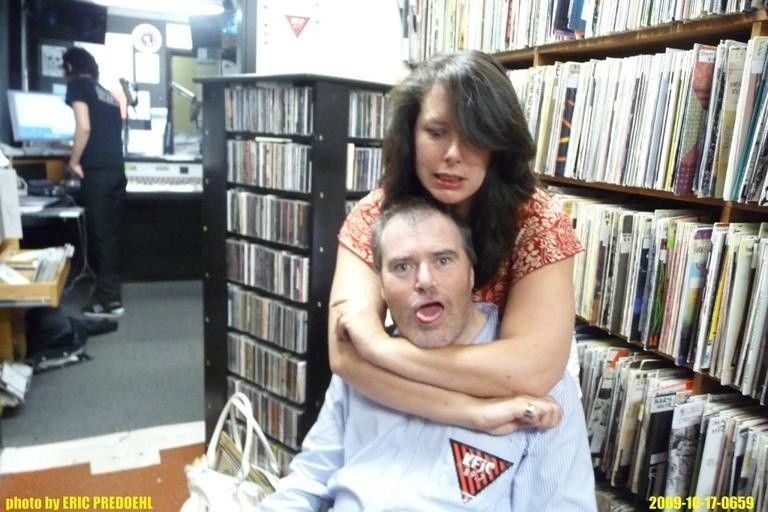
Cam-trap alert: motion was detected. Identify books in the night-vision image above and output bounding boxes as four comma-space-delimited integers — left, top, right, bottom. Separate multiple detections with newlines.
226, 78, 317, 479
405, 0, 768, 511
8, 250, 42, 270
345, 90, 390, 213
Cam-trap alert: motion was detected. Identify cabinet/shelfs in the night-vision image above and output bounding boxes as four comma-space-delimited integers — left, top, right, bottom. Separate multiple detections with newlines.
193, 73, 394, 476
407, 8, 768, 512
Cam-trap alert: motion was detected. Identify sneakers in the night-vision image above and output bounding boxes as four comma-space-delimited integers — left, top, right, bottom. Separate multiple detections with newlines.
82, 301, 124, 317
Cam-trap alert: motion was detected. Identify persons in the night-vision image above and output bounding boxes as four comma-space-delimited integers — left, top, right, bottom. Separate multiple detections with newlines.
63, 47, 127, 319
327, 48, 587, 434
258, 197, 597, 511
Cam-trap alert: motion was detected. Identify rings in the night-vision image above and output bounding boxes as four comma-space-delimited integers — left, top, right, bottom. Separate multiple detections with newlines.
524, 404, 534, 419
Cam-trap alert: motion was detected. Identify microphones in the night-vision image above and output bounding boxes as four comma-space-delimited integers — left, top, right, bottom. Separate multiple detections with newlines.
169, 80, 197, 102
119, 78, 138, 107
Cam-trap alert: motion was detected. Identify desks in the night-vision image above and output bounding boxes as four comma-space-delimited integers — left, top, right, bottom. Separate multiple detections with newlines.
13, 158, 67, 182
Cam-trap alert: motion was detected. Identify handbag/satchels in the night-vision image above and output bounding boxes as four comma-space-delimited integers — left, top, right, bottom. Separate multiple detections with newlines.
175, 391, 282, 512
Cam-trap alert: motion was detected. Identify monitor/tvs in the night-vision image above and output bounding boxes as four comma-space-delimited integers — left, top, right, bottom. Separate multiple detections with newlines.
7, 89, 76, 155
